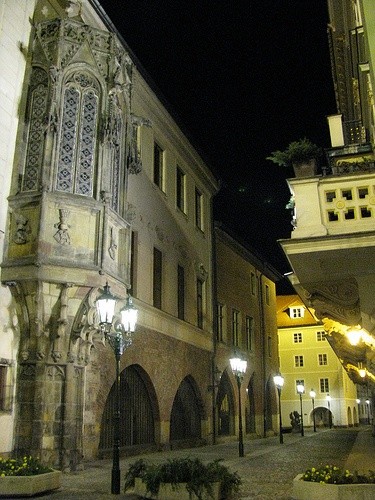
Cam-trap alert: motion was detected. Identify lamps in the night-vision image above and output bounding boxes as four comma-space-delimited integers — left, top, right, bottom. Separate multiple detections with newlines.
356, 354, 366, 370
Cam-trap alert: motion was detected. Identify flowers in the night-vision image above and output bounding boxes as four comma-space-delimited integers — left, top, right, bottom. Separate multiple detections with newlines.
0, 454, 53, 480
297, 462, 375, 484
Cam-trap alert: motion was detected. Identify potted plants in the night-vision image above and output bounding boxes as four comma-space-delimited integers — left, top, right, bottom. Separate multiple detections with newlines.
122, 455, 243, 500
266, 136, 321, 178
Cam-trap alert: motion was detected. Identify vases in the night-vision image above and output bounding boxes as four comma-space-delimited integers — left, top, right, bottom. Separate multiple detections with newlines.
293, 473, 375, 500
0, 467, 61, 496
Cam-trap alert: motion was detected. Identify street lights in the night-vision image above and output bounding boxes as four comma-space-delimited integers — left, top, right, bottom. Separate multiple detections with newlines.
229, 350, 248, 458
309, 388, 317, 432
272, 371, 285, 445
326, 394, 332, 429
365, 399, 371, 425
296, 382, 305, 437
95, 281, 139, 494
356, 398, 361, 426
